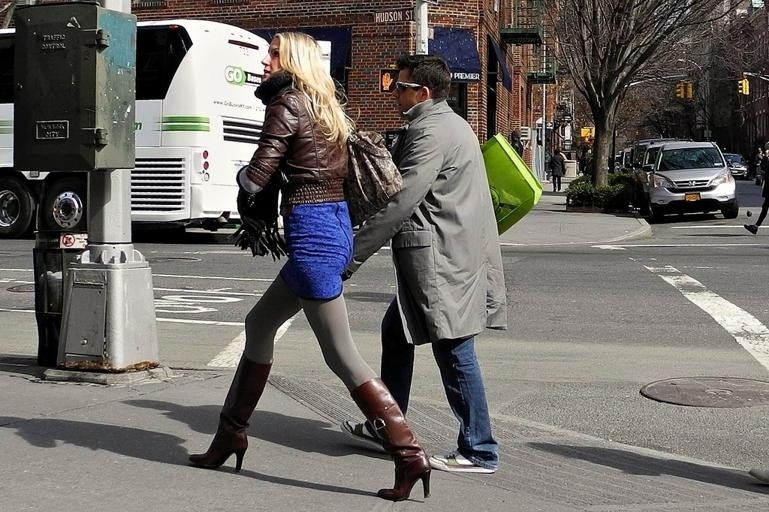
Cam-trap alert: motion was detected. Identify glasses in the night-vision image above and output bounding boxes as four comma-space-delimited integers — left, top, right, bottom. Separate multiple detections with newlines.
395, 80, 424, 93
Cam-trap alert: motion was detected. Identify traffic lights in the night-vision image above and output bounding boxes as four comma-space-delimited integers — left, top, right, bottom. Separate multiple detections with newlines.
738, 80, 750, 95
676, 83, 684, 99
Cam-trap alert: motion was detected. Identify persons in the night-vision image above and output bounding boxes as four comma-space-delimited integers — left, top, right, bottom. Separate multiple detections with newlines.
743, 141, 768, 235
549, 149, 566, 192
189, 28, 431, 501
341, 53, 508, 471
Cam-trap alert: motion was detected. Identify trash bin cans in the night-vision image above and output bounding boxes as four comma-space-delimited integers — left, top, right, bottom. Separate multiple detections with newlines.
33, 231, 89, 368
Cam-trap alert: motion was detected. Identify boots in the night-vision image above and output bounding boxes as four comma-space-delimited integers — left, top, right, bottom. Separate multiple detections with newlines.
338, 376, 432, 502
186, 351, 275, 475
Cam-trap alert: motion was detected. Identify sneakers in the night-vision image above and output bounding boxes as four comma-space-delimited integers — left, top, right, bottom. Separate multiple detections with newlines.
424, 447, 499, 477
339, 416, 391, 455
553, 188, 561, 192
743, 224, 759, 235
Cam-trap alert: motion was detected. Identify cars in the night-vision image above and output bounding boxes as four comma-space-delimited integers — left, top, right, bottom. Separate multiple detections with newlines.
613, 139, 749, 221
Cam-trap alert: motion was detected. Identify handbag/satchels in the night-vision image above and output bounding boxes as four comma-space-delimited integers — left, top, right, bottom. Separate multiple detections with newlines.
343, 128, 409, 230
479, 131, 546, 237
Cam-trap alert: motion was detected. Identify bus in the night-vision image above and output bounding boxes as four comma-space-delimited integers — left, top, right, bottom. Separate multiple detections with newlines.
2, 18, 283, 239
